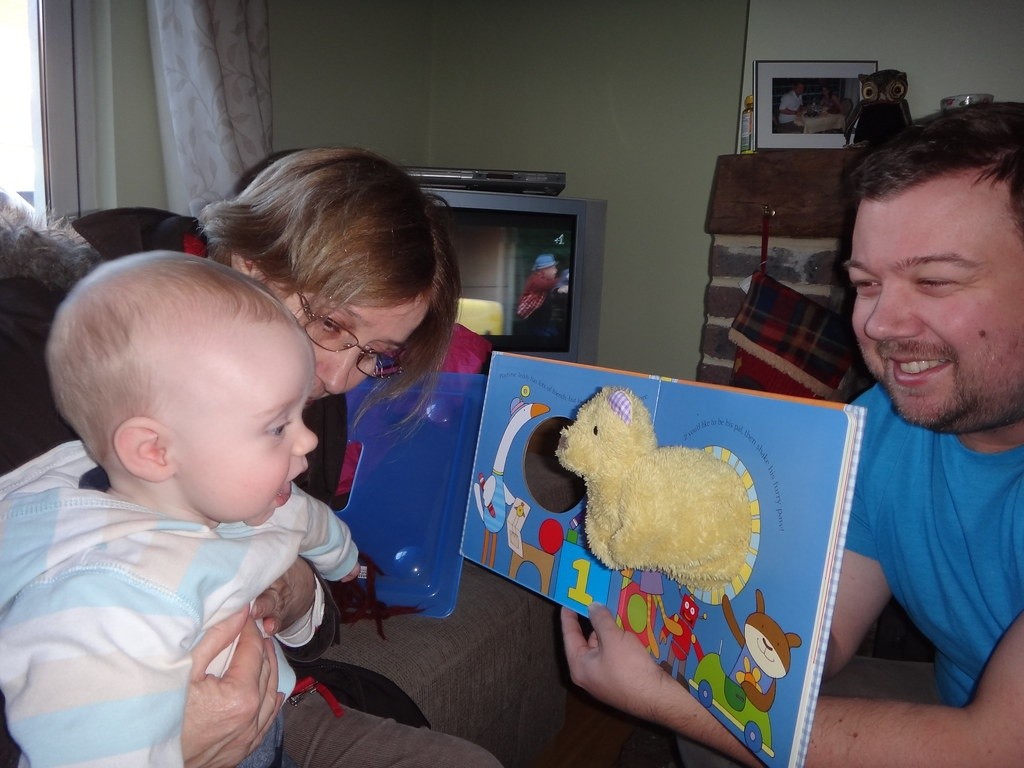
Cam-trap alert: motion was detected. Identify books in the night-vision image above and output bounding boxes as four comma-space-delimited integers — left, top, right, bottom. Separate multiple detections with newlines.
460, 351, 867, 768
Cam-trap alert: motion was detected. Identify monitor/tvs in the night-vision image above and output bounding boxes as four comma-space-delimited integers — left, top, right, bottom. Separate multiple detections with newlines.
417, 188, 608, 376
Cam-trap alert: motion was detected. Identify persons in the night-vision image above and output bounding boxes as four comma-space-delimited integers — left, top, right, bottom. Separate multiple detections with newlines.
0, 250, 507, 768
516, 254, 569, 337
562, 103, 1024, 768
778, 83, 804, 130
820, 86, 843, 110
0, 146, 460, 768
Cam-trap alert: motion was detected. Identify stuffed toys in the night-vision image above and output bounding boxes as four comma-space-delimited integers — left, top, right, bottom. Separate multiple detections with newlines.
556, 384, 751, 590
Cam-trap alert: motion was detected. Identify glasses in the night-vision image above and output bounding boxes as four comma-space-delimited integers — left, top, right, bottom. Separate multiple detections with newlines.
287, 251, 406, 379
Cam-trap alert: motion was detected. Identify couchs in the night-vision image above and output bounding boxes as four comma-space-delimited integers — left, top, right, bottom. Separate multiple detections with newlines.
320, 558, 569, 767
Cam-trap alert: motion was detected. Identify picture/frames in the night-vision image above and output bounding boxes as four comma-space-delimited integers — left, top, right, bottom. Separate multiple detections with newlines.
752, 59, 878, 152
